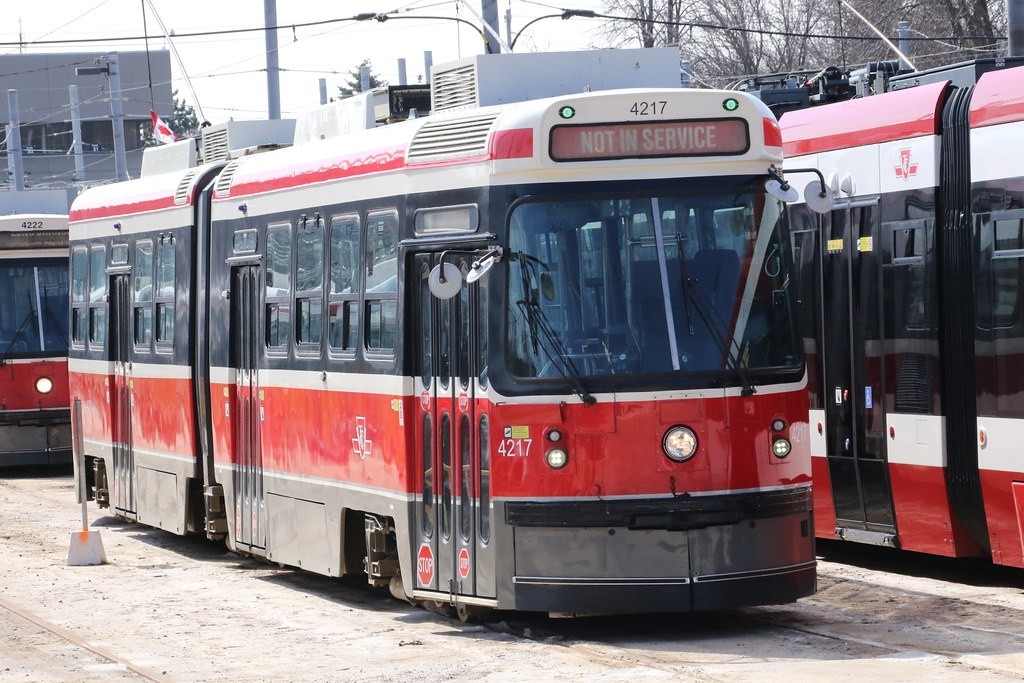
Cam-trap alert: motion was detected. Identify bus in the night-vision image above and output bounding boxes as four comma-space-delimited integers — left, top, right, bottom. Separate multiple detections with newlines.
715, 33, 1024, 578
66, 1, 837, 627
0, 212, 73, 473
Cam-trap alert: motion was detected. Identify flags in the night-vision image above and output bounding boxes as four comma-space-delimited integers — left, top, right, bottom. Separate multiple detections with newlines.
150, 108, 178, 144
